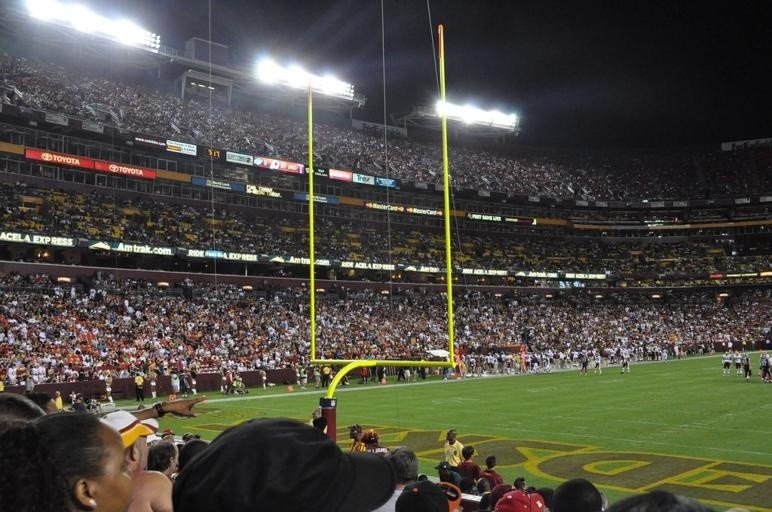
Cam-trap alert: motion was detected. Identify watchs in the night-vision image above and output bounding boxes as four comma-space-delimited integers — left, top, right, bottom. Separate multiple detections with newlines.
153, 401, 168, 418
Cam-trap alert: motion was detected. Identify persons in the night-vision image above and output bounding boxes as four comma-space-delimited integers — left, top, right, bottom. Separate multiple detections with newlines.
741, 353, 752, 384
593, 353, 602, 374
733, 351, 742, 376
620, 351, 630, 374
2, 48, 771, 198
581, 353, 589, 376
2, 392, 702, 510
4, 193, 770, 398
721, 351, 732, 376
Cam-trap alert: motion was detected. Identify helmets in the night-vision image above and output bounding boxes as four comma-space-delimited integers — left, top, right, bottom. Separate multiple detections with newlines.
361, 430, 379, 444
348, 424, 361, 432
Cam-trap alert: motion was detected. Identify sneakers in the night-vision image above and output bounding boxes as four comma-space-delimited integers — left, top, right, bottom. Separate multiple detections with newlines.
620, 369, 630, 374
761, 377, 772, 382
744, 377, 749, 383
722, 372, 743, 376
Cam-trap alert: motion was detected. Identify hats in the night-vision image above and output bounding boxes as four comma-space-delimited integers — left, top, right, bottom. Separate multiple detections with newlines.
435, 462, 452, 470
99, 410, 175, 449
396, 480, 461, 512
172, 417, 394, 512
494, 490, 544, 512
550, 479, 602, 512
